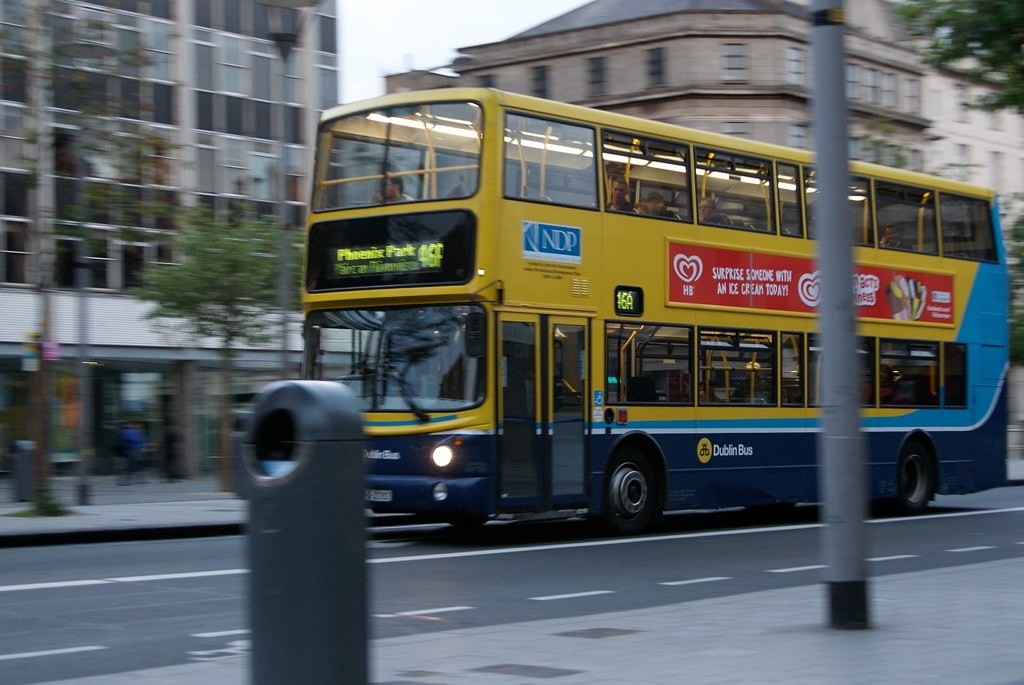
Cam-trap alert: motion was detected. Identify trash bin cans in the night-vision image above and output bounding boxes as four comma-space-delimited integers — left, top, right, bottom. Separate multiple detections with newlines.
12, 440, 36, 501
238, 378, 369, 685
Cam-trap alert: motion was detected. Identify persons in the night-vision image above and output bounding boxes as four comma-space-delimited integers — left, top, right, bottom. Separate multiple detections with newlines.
639, 191, 673, 217
159, 421, 179, 480
375, 166, 415, 202
880, 223, 912, 249
120, 419, 148, 485
748, 378, 772, 403
699, 380, 719, 402
699, 197, 715, 219
608, 178, 639, 213
880, 364, 898, 405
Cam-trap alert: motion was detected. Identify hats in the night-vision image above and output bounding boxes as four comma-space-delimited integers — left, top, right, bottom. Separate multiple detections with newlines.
647, 191, 664, 202
745, 360, 760, 371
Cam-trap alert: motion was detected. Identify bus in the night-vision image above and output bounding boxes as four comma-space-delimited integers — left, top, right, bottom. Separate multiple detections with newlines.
297, 88, 1010, 539
